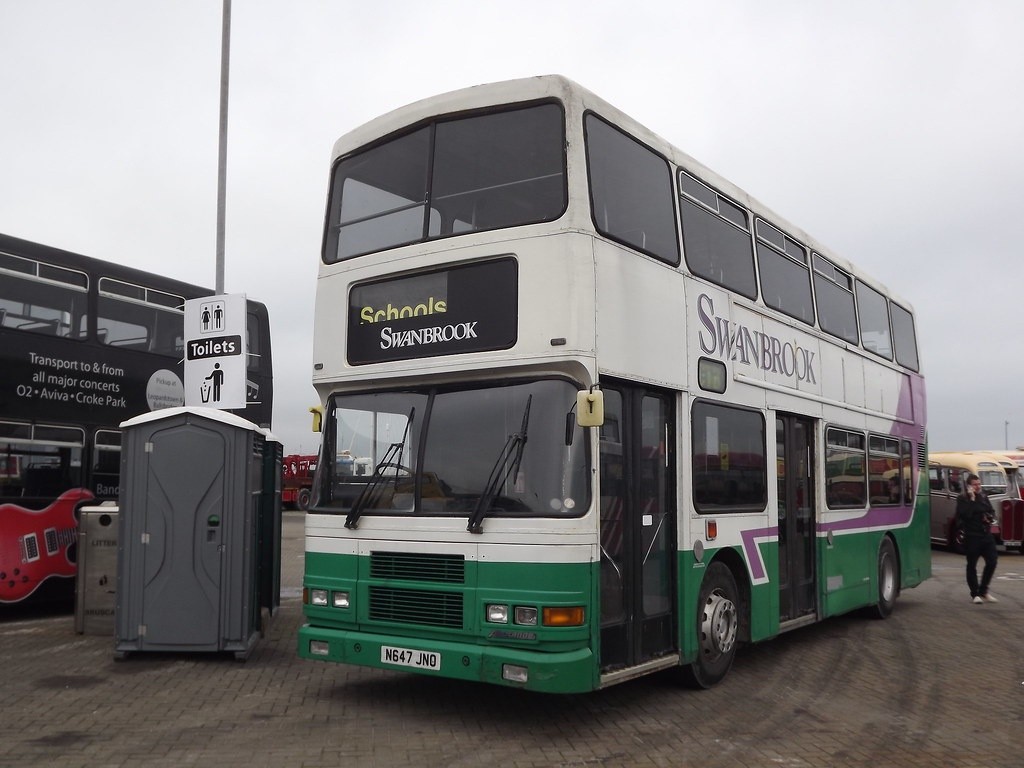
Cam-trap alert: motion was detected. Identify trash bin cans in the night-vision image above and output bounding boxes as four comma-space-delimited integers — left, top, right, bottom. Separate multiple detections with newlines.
76, 507, 125, 637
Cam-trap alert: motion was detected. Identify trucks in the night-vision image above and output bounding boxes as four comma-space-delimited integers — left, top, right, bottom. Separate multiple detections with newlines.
282, 449, 354, 510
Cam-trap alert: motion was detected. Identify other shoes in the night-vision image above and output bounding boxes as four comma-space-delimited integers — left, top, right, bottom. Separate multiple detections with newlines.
973, 596, 984, 603
978, 590, 998, 602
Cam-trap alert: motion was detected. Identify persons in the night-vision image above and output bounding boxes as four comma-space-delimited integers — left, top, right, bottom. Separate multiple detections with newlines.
955, 474, 999, 603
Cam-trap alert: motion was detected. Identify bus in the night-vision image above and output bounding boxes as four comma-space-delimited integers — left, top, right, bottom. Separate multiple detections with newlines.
295, 75, 931, 693
0, 233, 272, 609
928, 450, 1024, 555
